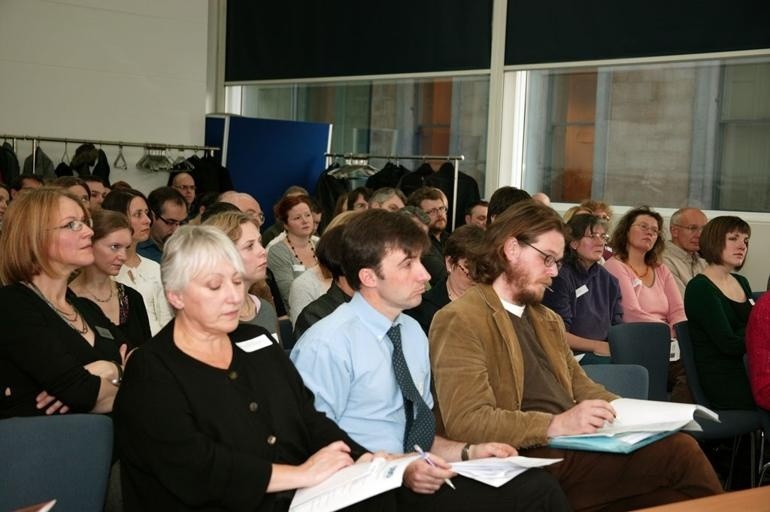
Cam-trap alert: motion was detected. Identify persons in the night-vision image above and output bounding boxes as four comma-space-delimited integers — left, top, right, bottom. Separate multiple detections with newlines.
1, 171, 770, 512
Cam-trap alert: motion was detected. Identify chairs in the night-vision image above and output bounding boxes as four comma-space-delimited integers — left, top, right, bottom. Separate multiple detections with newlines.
1, 415, 112, 511
674, 319, 715, 410
606, 322, 770, 490
577, 364, 651, 406
752, 291, 763, 304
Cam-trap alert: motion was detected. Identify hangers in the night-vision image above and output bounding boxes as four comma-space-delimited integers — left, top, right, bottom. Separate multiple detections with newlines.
61, 138, 70, 167
4, 134, 7, 140
386, 153, 392, 165
447, 155, 449, 163
100, 142, 102, 150
396, 152, 400, 167
36, 136, 40, 147
114, 144, 128, 170
325, 151, 381, 180
135, 143, 218, 175
422, 153, 428, 165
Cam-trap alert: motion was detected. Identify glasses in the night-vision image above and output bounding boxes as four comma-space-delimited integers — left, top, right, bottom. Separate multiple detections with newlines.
423, 206, 449, 216
454, 260, 474, 279
577, 233, 610, 241
671, 223, 705, 232
149, 206, 191, 226
42, 215, 92, 233
629, 221, 659, 234
517, 236, 563, 269
172, 183, 195, 190
247, 209, 265, 225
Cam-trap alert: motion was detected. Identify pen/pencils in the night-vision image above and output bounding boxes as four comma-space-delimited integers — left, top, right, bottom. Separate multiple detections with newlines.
572, 399, 620, 421
414, 444, 456, 490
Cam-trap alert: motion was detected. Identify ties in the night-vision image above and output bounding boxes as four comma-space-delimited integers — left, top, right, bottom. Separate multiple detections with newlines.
386, 323, 438, 456
